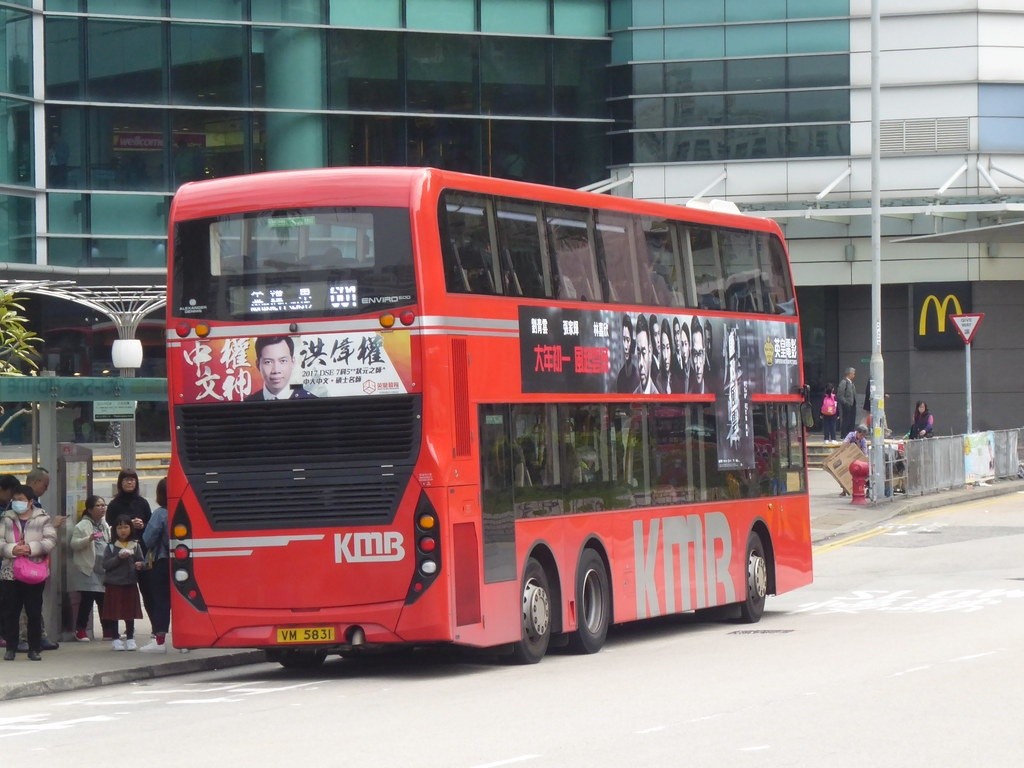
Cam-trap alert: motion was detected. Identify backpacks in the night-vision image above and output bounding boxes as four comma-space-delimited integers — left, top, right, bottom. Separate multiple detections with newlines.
821, 394, 836, 416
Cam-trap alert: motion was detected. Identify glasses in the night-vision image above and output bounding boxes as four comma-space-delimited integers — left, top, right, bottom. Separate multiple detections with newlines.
95, 504, 107, 508
691, 349, 704, 363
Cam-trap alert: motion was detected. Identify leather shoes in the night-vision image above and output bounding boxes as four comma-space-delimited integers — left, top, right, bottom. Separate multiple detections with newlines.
4, 650, 41, 660
17, 637, 59, 652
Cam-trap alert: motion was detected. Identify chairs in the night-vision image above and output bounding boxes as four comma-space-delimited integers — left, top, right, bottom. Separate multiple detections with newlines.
214, 241, 790, 318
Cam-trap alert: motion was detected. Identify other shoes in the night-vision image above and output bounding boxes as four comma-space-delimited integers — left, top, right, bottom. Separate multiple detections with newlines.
825, 440, 838, 444
839, 492, 846, 496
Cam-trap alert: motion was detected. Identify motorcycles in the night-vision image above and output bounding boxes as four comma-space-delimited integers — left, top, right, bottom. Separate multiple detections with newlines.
107, 421, 122, 448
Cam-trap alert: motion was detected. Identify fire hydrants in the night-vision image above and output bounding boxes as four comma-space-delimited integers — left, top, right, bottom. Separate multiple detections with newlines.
849, 458, 871, 505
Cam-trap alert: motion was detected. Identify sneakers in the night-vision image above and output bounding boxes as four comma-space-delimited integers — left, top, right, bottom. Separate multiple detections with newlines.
102, 631, 127, 641
74, 629, 90, 642
112, 639, 137, 650
139, 640, 167, 653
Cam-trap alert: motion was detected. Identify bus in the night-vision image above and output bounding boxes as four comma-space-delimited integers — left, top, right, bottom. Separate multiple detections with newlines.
166, 164, 817, 668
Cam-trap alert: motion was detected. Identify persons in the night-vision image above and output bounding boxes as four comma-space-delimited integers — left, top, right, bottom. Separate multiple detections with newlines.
0, 473, 22, 513
244, 335, 322, 402
0, 486, 58, 662
912, 400, 935, 439
863, 375, 890, 436
820, 383, 841, 445
14, 467, 68, 653
838, 424, 870, 496
69, 494, 124, 643
615, 313, 720, 395
139, 477, 171, 653
104, 468, 159, 639
516, 410, 690, 487
835, 368, 858, 441
101, 513, 148, 651
908, 424, 920, 441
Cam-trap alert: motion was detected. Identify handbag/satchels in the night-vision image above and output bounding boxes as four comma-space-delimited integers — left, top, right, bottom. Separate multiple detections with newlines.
13, 555, 48, 584
910, 424, 919, 439
